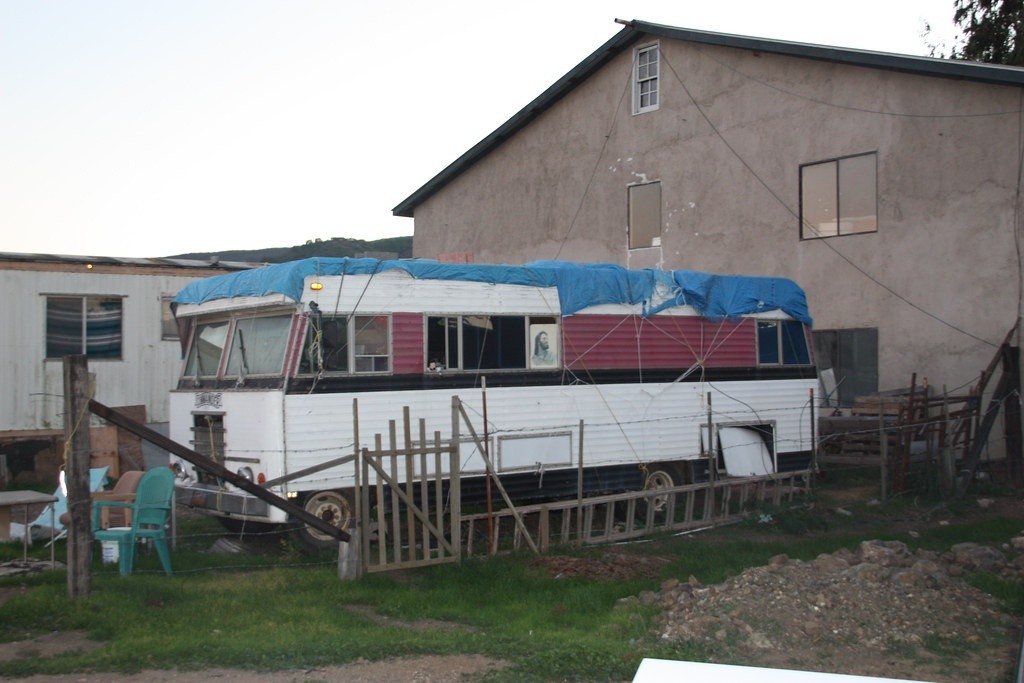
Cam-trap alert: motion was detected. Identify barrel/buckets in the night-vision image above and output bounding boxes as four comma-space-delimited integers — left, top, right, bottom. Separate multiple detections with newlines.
100, 526, 132, 563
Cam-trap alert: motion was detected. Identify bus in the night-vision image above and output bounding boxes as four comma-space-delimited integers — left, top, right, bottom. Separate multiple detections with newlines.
168, 257, 822, 563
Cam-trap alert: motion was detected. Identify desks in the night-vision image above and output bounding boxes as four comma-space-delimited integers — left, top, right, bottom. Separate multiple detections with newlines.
0, 489, 59, 576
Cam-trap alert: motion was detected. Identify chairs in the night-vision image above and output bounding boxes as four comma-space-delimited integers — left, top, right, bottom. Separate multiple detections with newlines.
90, 466, 175, 576
91, 469, 143, 529
